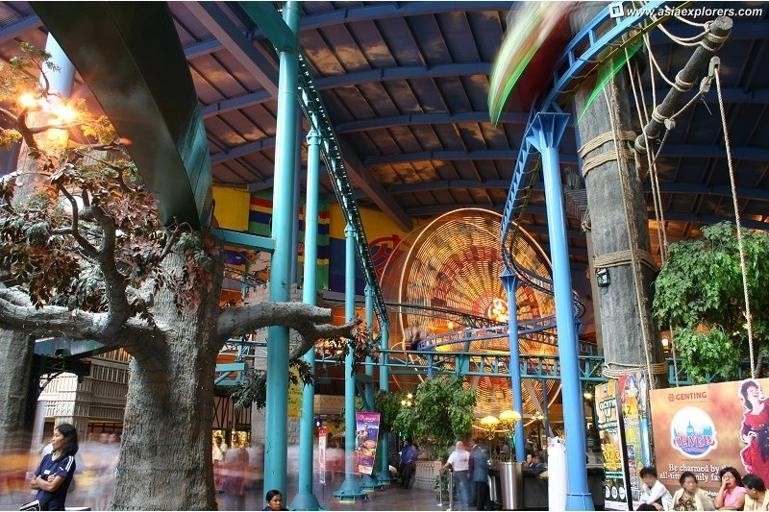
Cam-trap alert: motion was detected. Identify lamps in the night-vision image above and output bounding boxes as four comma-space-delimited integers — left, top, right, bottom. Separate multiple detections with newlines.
479, 408, 522, 464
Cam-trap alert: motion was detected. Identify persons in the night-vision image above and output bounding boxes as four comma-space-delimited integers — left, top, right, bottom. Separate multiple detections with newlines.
86, 432, 120, 511
739, 380, 769, 490
441, 437, 491, 510
397, 437, 418, 489
635, 466, 674, 511
671, 471, 716, 511
212, 432, 249, 510
28, 423, 79, 511
501, 441, 547, 475
713, 467, 747, 511
741, 473, 769, 511
260, 489, 290, 511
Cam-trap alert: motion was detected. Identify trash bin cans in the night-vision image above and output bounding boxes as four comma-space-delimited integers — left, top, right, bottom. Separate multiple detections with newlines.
500, 462, 523, 510
